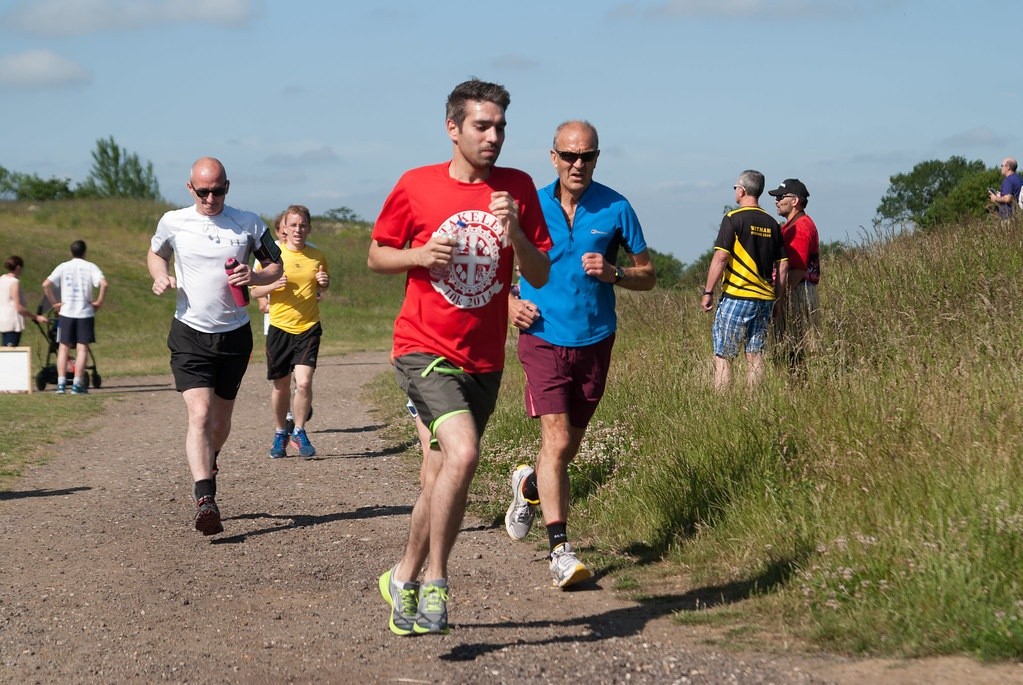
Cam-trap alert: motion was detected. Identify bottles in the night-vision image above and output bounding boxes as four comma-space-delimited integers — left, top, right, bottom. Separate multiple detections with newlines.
226, 258, 249, 306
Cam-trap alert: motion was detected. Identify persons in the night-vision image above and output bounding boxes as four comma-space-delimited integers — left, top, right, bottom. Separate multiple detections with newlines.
247, 205, 330, 460
42, 240, 107, 394
147, 157, 284, 536
504, 120, 656, 589
0, 256, 47, 346
253, 213, 320, 435
768, 178, 821, 367
990, 158, 1023, 230
366, 80, 555, 635
700, 170, 789, 395
512, 265, 521, 299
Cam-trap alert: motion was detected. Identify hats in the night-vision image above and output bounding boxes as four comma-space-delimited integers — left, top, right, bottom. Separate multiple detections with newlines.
768, 179, 809, 198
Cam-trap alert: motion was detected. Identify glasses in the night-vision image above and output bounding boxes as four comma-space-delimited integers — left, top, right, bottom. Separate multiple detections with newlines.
733, 185, 745, 191
190, 181, 226, 198
555, 150, 600, 162
777, 194, 800, 201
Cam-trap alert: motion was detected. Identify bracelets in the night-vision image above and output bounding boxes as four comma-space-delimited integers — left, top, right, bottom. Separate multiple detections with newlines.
703, 290, 714, 295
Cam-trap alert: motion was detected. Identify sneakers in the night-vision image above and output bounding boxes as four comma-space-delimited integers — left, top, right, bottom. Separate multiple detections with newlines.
193, 493, 223, 536
548, 542, 589, 587
505, 464, 541, 541
55, 384, 64, 393
70, 385, 87, 394
290, 429, 315, 457
378, 563, 421, 635
190, 463, 218, 502
413, 578, 451, 634
270, 427, 290, 458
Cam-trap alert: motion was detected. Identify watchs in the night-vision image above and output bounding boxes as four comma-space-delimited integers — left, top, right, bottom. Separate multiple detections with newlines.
613, 266, 625, 284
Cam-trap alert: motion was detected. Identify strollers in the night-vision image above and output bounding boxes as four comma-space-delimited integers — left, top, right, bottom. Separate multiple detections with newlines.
32, 299, 104, 390
